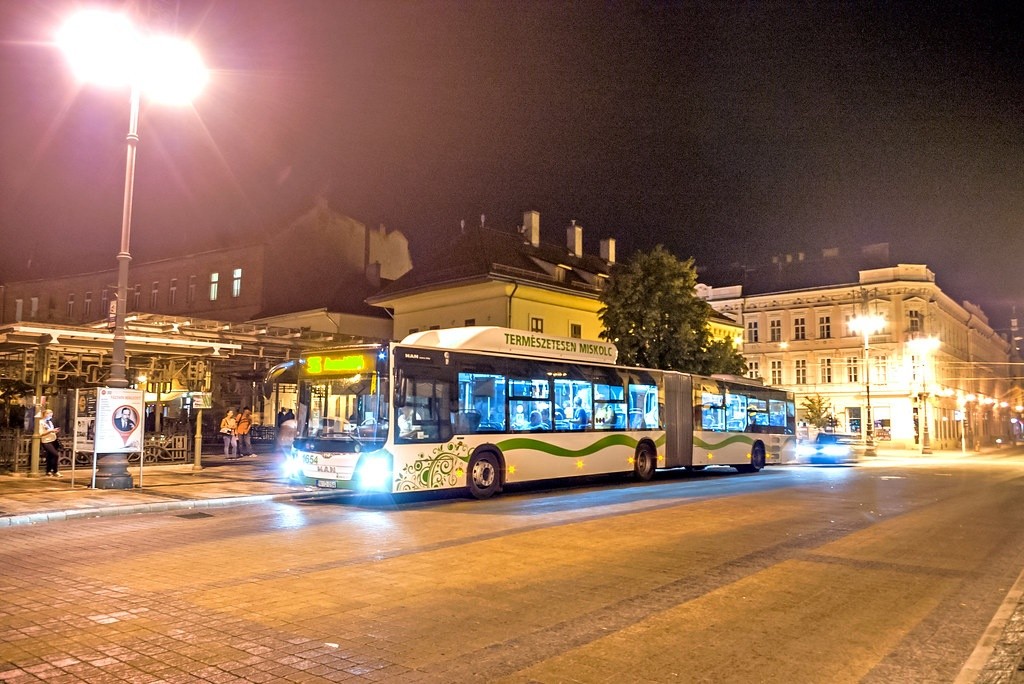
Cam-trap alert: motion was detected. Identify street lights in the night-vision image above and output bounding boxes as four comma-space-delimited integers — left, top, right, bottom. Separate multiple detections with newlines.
848, 314, 887, 457
52, 8, 210, 491
908, 337, 939, 454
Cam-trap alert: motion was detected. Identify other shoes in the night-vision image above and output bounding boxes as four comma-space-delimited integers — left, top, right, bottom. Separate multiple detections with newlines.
46, 472, 53, 477
249, 453, 257, 457
226, 457, 237, 461
53, 472, 63, 478
238, 455, 244, 460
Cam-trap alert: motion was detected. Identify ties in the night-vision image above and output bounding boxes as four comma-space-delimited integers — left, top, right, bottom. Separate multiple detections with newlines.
124, 422, 126, 428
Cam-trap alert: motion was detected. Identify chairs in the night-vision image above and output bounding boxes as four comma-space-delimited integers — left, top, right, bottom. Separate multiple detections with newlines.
530, 408, 643, 430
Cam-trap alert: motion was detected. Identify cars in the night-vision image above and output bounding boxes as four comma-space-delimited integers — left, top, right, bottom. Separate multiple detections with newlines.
806, 431, 858, 465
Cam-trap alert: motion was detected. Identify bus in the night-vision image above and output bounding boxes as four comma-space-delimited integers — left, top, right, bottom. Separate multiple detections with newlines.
262, 324, 798, 501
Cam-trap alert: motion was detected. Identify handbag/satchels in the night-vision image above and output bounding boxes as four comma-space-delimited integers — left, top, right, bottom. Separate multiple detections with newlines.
220, 418, 231, 435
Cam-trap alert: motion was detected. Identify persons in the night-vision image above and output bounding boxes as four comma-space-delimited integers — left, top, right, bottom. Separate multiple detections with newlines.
278, 407, 286, 428
114, 408, 135, 431
780, 405, 788, 414
38, 409, 64, 479
236, 406, 257, 457
285, 408, 295, 421
221, 410, 239, 460
521, 394, 614, 431
398, 402, 422, 439
